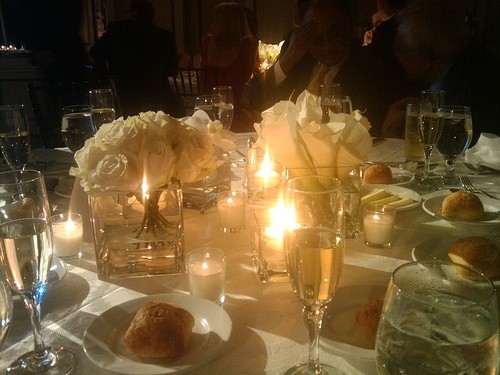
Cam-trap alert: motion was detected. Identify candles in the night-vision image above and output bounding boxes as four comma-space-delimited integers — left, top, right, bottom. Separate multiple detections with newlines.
217, 191, 245, 230
188, 249, 225, 303
257, 170, 278, 186
364, 202, 396, 249
50, 213, 83, 256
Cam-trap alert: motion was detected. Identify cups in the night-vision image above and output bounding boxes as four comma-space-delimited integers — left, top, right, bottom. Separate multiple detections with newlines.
215, 191, 246, 233
88, 178, 185, 280
61, 88, 115, 153
321, 82, 352, 124
186, 247, 226, 308
50, 212, 84, 261
244, 148, 286, 204
404, 102, 431, 167
194, 86, 234, 130
363, 202, 396, 249
375, 259, 500, 375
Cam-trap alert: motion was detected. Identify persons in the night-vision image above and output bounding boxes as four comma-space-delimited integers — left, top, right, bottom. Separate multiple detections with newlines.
252, 0, 414, 137
15, 0, 87, 63
363, 0, 500, 147
90, 0, 178, 102
197, 3, 260, 103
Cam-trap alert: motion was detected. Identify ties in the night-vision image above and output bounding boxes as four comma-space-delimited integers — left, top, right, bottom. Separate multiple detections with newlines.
317, 61, 330, 96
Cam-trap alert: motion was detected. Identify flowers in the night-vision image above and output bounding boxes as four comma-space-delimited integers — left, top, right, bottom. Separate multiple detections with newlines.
67, 105, 233, 248
256, 92, 377, 227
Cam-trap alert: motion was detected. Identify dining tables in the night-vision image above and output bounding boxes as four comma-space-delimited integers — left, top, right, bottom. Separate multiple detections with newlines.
0, 136, 500, 375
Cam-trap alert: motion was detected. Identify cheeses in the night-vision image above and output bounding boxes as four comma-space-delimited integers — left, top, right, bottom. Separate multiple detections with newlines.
361, 189, 414, 207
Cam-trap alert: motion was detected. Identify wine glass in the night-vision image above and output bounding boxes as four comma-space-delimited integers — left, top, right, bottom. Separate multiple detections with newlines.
421, 89, 473, 186
0, 170, 75, 375
283, 176, 346, 375
0, 102, 32, 198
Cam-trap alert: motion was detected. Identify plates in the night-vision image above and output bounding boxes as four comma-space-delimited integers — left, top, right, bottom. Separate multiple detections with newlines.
422, 195, 500, 226
81, 293, 234, 375
359, 183, 422, 212
387, 167, 415, 186
54, 178, 76, 199
411, 236, 500, 287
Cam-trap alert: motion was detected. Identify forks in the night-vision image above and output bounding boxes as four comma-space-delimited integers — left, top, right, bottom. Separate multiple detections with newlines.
457, 175, 500, 200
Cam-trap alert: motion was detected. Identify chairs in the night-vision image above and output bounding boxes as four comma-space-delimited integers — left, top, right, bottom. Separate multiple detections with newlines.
27, 81, 131, 147
163, 64, 219, 111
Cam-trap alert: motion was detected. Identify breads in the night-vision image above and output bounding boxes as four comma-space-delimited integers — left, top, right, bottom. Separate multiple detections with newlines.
448, 237, 500, 280
363, 164, 392, 184
124, 300, 194, 361
355, 300, 383, 343
441, 190, 484, 220
